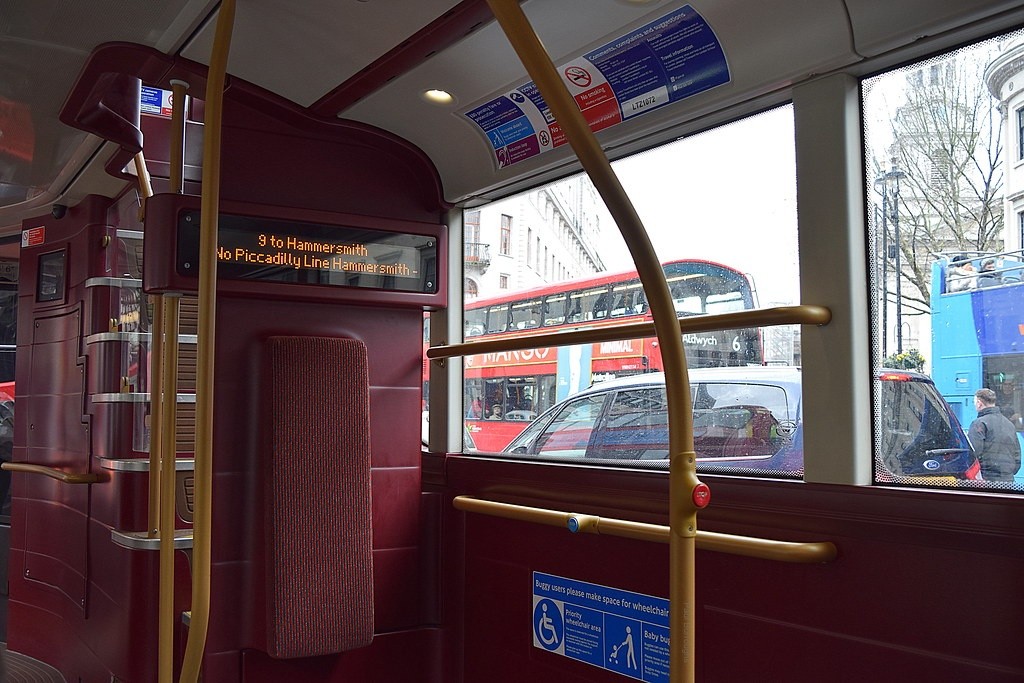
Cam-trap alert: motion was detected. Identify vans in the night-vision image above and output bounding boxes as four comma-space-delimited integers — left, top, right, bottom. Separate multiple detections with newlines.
500, 365, 985, 489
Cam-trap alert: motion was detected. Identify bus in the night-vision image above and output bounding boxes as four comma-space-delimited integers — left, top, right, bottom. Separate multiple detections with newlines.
931, 249, 1024, 487
422, 260, 764, 459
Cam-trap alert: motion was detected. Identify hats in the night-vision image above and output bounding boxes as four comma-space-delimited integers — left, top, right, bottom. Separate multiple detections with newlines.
492, 404, 501, 408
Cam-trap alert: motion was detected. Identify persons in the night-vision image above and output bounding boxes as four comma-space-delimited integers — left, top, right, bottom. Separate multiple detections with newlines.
966, 389, 1022, 482
994, 374, 1024, 430
489, 404, 501, 419
467, 394, 490, 418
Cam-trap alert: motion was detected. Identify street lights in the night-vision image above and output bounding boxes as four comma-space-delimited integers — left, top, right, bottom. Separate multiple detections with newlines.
873, 154, 906, 365
